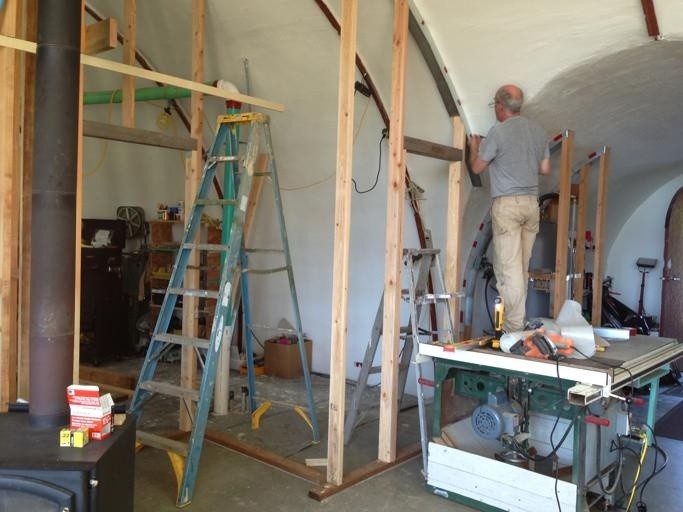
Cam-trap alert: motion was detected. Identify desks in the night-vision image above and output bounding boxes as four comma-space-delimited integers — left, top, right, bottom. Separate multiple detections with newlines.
0, 410, 139, 511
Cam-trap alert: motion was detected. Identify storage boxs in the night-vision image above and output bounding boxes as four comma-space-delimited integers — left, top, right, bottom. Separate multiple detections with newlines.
264, 338, 312, 378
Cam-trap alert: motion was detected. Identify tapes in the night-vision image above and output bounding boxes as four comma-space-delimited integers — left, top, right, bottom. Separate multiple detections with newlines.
623, 327, 637, 336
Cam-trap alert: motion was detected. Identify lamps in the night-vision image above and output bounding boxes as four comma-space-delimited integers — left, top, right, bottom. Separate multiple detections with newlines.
157, 104, 172, 130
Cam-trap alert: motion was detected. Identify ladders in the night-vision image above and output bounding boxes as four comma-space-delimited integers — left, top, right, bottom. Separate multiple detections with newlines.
344, 248, 457, 482
129, 112, 320, 508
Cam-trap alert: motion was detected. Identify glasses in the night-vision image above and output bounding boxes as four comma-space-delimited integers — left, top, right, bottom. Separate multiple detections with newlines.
487, 101, 501, 109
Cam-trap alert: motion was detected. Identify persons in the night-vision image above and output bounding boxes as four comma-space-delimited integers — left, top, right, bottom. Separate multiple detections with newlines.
469, 84, 550, 331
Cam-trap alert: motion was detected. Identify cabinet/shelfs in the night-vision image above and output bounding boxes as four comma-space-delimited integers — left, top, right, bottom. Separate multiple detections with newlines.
80, 218, 126, 351
147, 219, 242, 354
